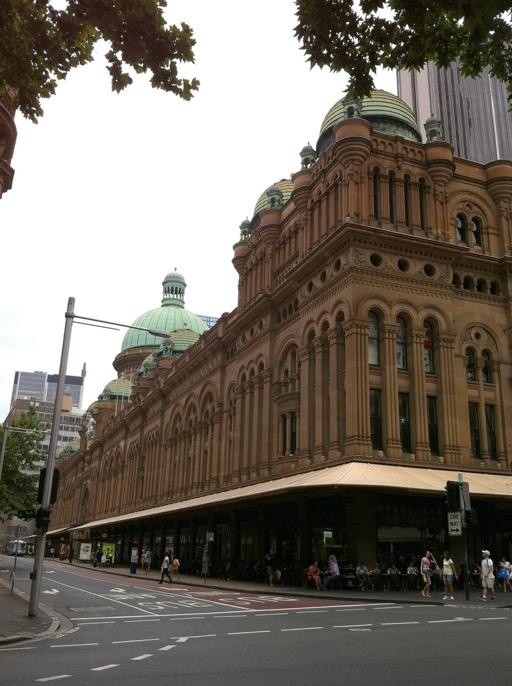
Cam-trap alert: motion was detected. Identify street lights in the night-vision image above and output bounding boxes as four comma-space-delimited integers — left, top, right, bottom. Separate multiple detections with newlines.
26, 292, 171, 617
0, 420, 60, 482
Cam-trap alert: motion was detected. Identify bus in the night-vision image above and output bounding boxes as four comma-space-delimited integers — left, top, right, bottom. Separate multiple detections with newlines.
7, 539, 27, 556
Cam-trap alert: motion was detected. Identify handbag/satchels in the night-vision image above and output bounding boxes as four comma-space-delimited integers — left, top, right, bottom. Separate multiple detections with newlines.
498, 567, 510, 577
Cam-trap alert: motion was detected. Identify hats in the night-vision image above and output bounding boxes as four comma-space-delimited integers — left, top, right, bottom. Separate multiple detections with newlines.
482, 549, 491, 556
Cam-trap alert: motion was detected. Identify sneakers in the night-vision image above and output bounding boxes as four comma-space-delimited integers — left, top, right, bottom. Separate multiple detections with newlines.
450, 595, 454, 600
441, 595, 449, 600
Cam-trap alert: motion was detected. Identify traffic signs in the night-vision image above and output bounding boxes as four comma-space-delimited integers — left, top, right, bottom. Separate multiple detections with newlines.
446, 511, 465, 537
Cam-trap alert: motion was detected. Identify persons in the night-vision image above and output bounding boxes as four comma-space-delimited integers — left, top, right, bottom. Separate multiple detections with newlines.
306, 549, 511, 603
264, 547, 280, 588
201, 550, 212, 578
46, 545, 182, 586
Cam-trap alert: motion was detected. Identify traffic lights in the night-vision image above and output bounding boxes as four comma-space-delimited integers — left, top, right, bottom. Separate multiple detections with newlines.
443, 480, 457, 509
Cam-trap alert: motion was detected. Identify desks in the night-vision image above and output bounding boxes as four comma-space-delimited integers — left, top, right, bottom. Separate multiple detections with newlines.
279, 567, 418, 593
